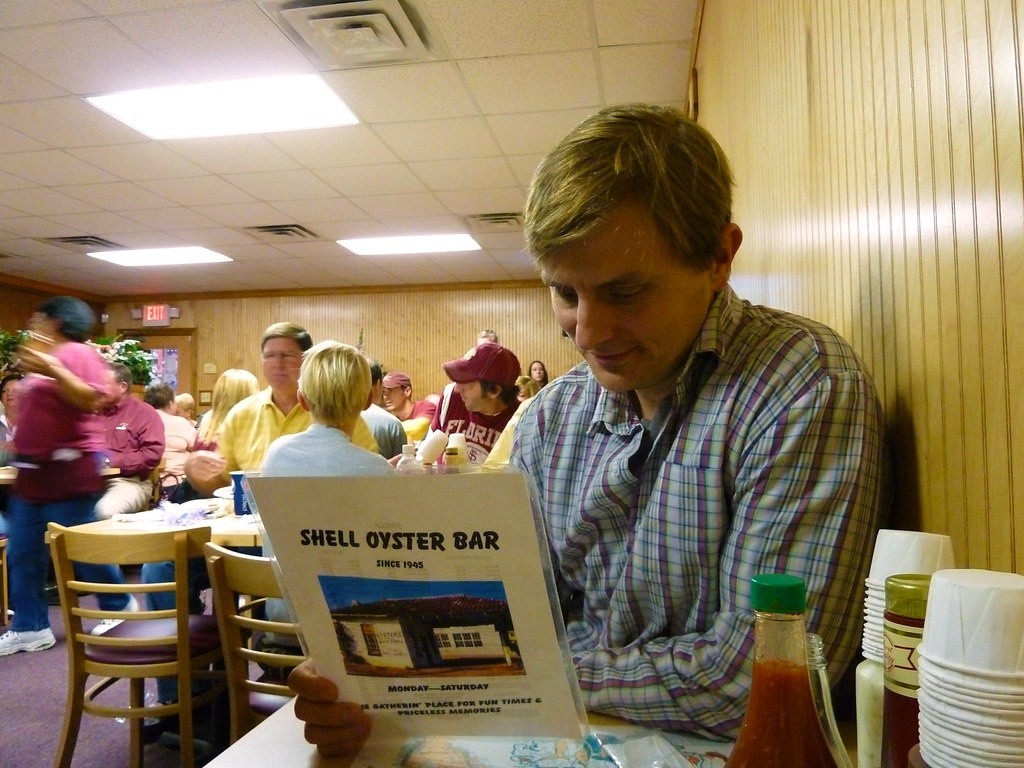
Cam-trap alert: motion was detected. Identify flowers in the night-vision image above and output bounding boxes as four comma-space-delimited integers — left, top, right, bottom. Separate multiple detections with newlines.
85, 333, 155, 384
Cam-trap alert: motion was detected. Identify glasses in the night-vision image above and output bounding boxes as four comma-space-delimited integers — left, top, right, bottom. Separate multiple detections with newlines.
261, 350, 301, 361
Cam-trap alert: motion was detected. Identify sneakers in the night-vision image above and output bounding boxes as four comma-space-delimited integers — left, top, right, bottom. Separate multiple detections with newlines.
91, 599, 138, 636
0, 627, 56, 656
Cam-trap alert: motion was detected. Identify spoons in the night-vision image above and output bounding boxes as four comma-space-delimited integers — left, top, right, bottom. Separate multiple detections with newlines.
623, 739, 658, 768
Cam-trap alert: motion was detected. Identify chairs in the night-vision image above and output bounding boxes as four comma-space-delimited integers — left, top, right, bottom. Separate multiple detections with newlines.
47, 521, 212, 768
204, 541, 310, 745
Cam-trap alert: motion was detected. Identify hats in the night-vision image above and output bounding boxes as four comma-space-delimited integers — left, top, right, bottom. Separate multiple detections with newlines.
381, 372, 411, 388
442, 343, 521, 387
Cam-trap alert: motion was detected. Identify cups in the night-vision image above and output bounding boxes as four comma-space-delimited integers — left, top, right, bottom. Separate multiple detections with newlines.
230, 473, 251, 515
861, 529, 955, 664
447, 433, 471, 472
915, 568, 1024, 768
416, 429, 448, 464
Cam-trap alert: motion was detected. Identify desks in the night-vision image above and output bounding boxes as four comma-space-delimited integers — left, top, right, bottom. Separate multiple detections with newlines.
44, 497, 264, 548
0, 466, 120, 484
201, 693, 735, 768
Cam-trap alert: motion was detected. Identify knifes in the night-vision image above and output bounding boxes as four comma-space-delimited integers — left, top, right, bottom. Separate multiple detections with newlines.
654, 735, 683, 768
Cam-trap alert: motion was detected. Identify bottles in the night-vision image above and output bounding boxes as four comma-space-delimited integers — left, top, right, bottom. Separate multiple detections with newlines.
805, 632, 853, 768
723, 572, 837, 768
396, 444, 422, 476
444, 446, 459, 466
879, 574, 932, 768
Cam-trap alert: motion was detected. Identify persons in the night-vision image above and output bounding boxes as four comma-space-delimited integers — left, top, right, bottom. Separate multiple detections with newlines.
142, 381, 195, 497
517, 361, 549, 403
0, 373, 24, 537
289, 102, 890, 758
0, 295, 140, 656
358, 359, 408, 461
253, 339, 397, 685
381, 372, 436, 442
192, 367, 260, 450
474, 330, 499, 348
390, 341, 522, 473
141, 322, 381, 718
94, 361, 165, 575
174, 391, 200, 429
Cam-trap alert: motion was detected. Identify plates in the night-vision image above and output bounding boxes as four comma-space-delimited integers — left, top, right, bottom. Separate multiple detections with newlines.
214, 487, 234, 499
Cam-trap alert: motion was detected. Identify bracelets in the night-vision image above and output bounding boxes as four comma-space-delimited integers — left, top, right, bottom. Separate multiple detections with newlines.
103, 457, 110, 468
